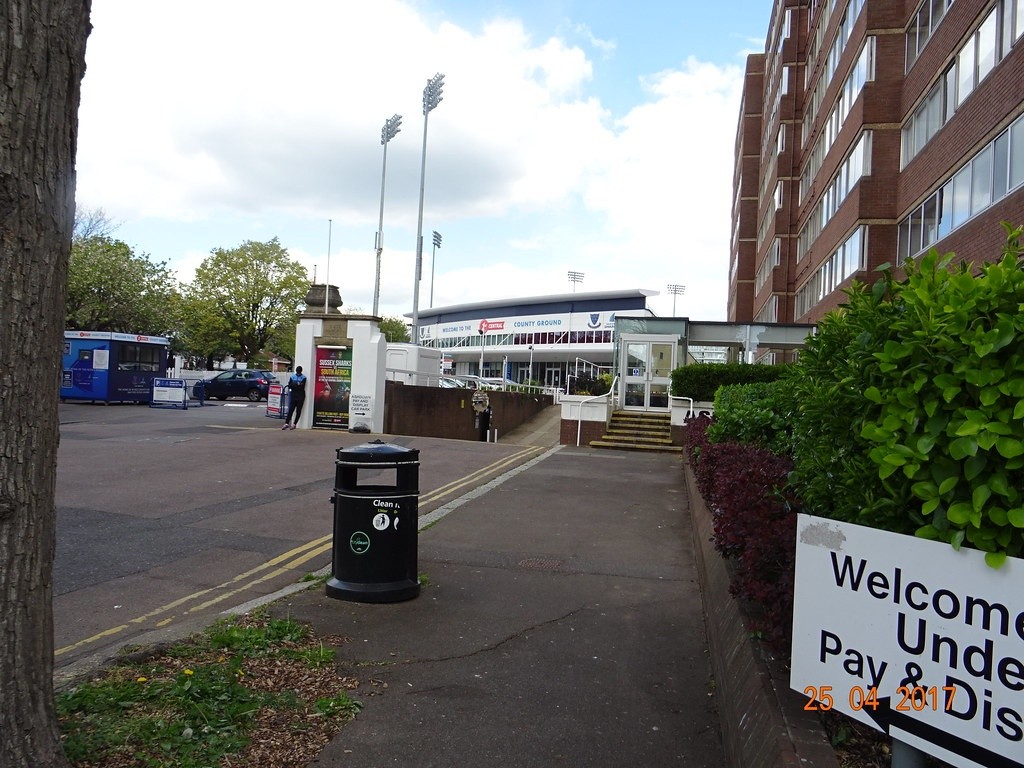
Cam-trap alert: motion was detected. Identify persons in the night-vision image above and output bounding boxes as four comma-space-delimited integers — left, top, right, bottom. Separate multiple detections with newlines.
281, 366, 306, 430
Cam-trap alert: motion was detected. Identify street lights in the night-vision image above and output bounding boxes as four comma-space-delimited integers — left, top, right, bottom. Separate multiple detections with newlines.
667, 283, 686, 318
429, 230, 442, 309
371, 112, 403, 318
409, 70, 445, 344
568, 270, 592, 293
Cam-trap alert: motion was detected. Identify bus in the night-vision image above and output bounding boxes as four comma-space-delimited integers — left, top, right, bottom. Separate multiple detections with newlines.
60, 331, 177, 405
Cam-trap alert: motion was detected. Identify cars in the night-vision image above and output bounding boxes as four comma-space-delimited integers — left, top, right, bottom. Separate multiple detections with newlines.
438, 372, 521, 392
193, 368, 280, 403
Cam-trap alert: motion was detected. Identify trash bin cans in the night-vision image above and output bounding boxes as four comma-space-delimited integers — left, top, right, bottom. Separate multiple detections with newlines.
326, 440, 421, 603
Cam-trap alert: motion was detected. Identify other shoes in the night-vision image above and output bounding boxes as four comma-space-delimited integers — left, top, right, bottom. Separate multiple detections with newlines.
282, 424, 289, 430
290, 425, 296, 430
